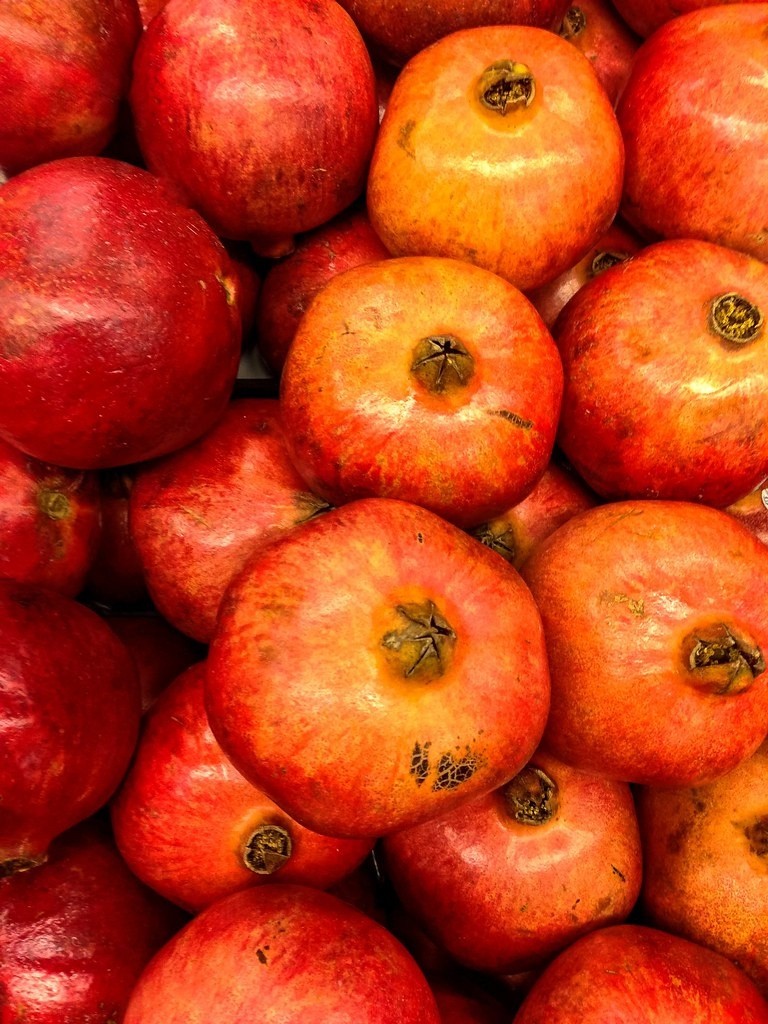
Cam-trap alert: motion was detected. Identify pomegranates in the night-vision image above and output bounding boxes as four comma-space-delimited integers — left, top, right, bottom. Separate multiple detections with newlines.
0, 0, 768, 1024
